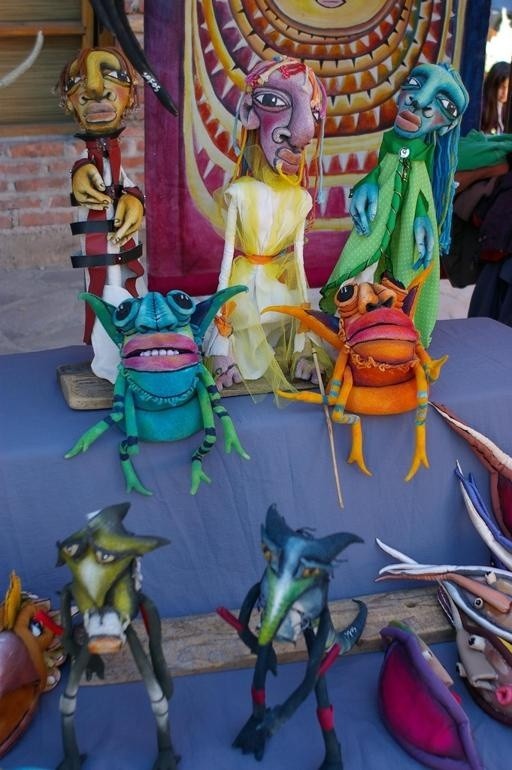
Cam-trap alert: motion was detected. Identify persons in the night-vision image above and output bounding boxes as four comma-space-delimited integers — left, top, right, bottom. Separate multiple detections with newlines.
481, 61, 512, 135
